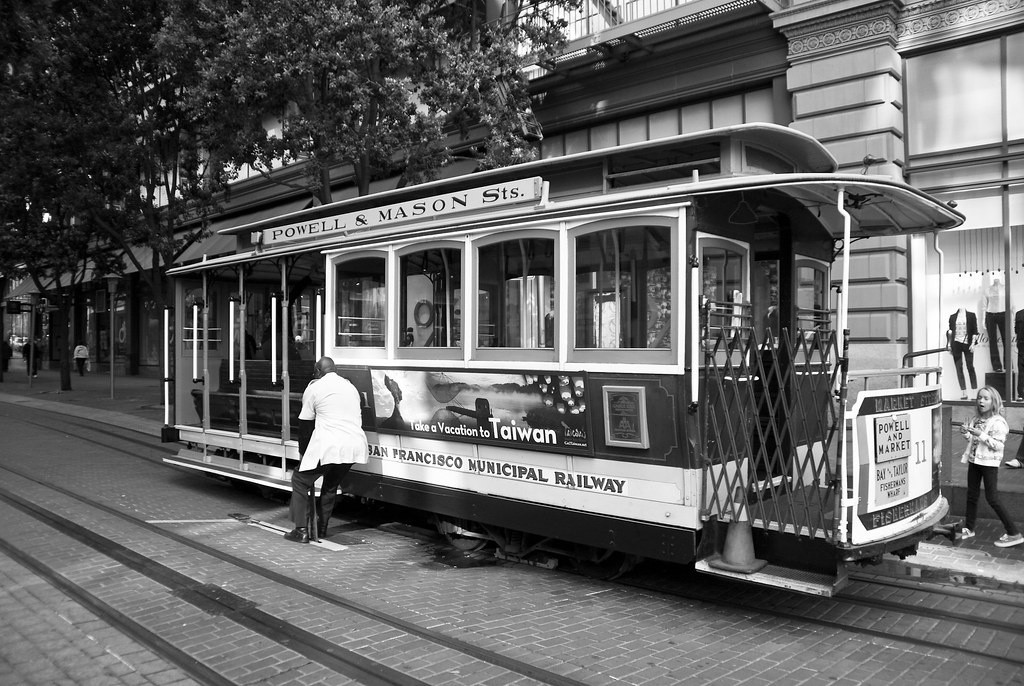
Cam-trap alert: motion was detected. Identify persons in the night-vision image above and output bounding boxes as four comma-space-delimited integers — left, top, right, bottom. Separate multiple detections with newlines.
1004, 428, 1024, 468
961, 386, 1024, 547
283, 357, 369, 543
982, 279, 1014, 372
4, 341, 13, 372
22, 338, 44, 378
74, 341, 89, 376
1014, 309, 1024, 401
948, 308, 979, 400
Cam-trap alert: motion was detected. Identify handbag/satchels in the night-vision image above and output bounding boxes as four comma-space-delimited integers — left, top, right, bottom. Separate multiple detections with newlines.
87, 361, 91, 372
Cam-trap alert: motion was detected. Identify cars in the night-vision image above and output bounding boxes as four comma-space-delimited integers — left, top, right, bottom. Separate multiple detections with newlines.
13, 336, 23, 351
10, 335, 16, 346
23, 337, 28, 347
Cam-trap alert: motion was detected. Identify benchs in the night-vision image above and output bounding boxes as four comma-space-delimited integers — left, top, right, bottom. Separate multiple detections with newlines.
192, 360, 313, 432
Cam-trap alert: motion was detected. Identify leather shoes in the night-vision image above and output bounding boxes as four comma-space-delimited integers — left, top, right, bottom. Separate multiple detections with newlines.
285, 527, 309, 542
316, 527, 328, 539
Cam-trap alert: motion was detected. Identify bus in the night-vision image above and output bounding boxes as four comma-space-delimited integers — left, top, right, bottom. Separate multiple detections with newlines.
164, 120, 966, 600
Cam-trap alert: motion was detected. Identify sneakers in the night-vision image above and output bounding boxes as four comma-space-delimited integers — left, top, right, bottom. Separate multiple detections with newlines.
961, 528, 975, 539
994, 533, 1024, 547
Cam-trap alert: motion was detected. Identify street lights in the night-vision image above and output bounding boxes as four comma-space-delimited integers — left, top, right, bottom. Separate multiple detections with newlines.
103, 273, 123, 400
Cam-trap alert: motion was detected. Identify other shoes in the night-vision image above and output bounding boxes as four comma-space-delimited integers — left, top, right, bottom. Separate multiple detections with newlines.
34, 374, 37, 378
1005, 458, 1024, 468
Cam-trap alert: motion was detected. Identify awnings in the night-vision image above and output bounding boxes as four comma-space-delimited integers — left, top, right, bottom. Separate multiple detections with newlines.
319, 159, 480, 206
173, 198, 312, 263
6, 228, 201, 298
